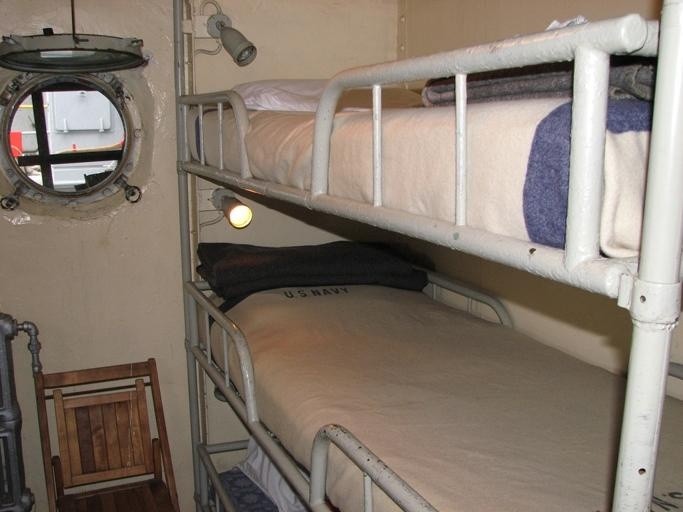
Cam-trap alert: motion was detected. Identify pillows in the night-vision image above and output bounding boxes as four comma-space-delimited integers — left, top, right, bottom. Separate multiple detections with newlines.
235, 78, 426, 113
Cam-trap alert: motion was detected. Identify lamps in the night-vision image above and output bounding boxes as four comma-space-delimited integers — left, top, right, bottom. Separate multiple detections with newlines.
206, 14, 258, 66
210, 187, 255, 232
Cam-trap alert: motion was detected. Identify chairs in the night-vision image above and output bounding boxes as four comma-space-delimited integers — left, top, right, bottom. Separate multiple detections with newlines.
31, 354, 186, 512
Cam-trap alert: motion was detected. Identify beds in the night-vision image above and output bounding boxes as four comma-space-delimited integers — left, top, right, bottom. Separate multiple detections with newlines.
175, 0, 682, 512
